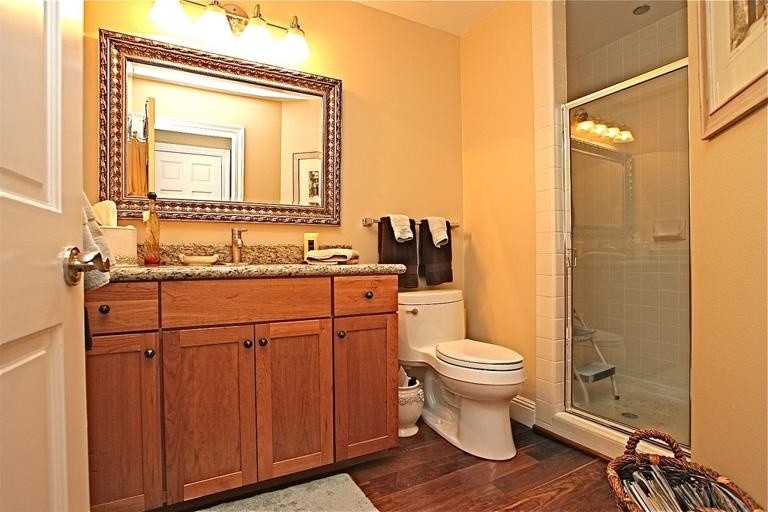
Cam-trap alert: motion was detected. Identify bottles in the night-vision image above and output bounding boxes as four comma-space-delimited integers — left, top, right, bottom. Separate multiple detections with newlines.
145, 192, 160, 266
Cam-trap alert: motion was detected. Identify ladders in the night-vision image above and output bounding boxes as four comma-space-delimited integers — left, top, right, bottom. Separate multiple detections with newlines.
570, 308, 622, 410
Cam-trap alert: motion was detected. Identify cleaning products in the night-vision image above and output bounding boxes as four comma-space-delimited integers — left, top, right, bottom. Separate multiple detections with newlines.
304, 232, 320, 258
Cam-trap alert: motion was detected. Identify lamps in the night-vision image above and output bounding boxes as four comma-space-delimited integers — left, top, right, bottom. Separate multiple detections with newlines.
149, 2, 308, 58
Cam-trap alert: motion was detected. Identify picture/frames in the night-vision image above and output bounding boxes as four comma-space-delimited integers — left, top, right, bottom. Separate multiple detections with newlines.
291, 152, 322, 205
698, 0, 768, 140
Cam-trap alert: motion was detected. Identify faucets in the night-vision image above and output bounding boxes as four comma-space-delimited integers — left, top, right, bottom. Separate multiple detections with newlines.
231, 228, 247, 264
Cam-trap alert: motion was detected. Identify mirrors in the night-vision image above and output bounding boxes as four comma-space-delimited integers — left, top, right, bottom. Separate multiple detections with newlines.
98, 30, 342, 225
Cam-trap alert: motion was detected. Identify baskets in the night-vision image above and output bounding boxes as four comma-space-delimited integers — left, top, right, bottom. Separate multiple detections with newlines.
605, 428, 768, 512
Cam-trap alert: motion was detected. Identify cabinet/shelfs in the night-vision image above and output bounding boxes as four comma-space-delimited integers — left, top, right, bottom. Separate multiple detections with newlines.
86, 276, 400, 512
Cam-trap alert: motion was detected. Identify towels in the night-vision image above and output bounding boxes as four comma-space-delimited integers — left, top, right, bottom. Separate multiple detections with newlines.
377, 216, 419, 289
418, 219, 453, 286
80, 189, 117, 293
420, 217, 449, 249
387, 214, 414, 243
305, 248, 361, 265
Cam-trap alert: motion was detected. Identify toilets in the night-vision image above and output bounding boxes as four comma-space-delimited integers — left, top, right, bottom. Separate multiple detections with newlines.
399, 290, 527, 461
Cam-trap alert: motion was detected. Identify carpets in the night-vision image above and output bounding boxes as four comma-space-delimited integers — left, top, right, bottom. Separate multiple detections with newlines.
194, 474, 382, 512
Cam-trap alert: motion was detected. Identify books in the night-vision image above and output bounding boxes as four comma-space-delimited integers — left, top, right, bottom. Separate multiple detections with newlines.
623, 464, 751, 512
